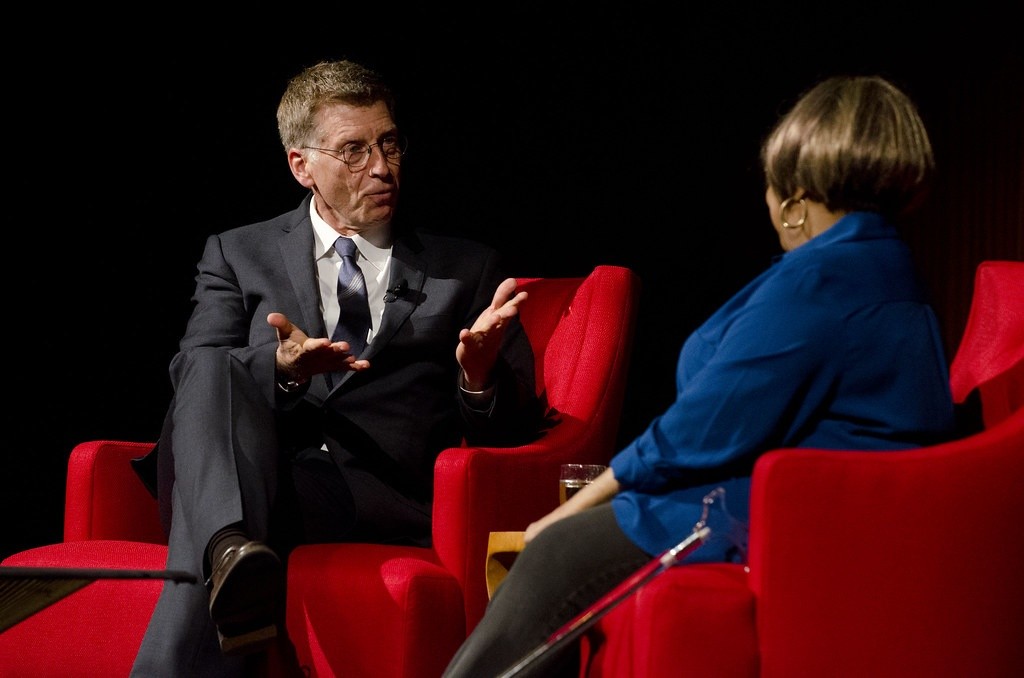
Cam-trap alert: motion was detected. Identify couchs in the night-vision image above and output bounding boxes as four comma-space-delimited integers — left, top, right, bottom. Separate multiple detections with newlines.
0, 259, 1023, 678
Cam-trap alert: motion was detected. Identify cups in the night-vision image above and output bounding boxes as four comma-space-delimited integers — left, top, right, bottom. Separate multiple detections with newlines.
560, 464, 606, 505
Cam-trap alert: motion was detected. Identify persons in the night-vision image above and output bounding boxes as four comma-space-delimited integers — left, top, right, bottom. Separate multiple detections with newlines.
439, 77, 955, 678
127, 59, 534, 678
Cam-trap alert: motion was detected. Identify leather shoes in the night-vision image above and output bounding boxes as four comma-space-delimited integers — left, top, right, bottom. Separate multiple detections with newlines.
206, 541, 282, 672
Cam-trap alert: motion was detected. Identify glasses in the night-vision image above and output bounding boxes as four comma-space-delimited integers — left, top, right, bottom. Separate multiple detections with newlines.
302, 134, 408, 173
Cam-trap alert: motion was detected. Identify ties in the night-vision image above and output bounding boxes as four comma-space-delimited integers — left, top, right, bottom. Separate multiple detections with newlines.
325, 236, 372, 391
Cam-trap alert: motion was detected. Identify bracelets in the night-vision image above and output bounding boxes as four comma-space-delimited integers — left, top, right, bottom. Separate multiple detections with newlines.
458, 373, 497, 400
